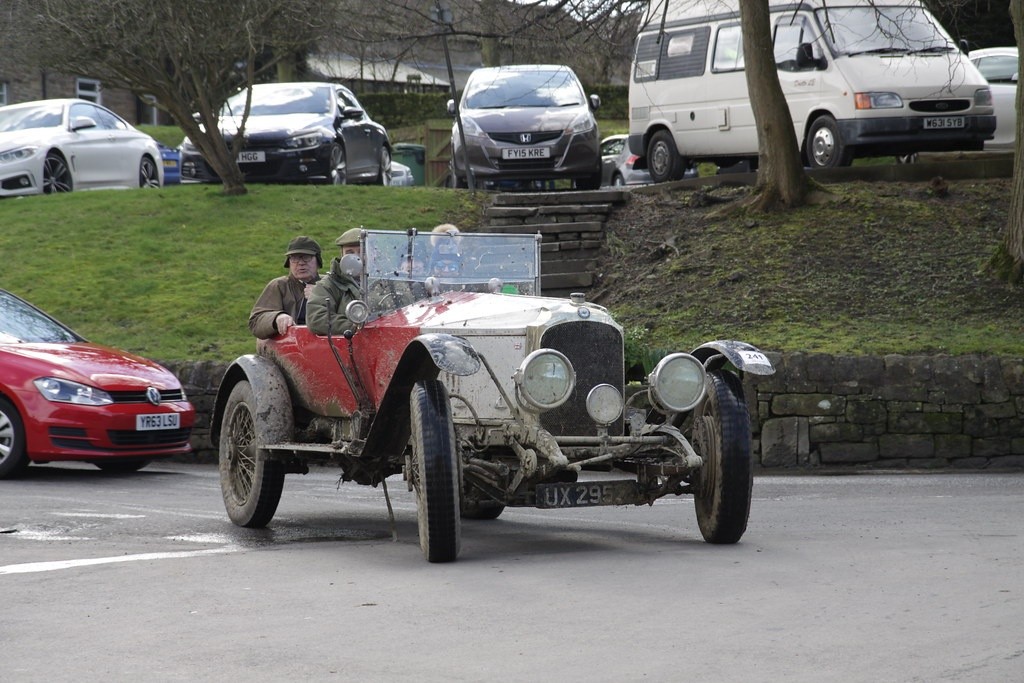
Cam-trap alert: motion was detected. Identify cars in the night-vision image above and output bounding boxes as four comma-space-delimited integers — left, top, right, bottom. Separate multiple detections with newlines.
207, 227, 775, 564
156, 139, 180, 187
569, 133, 699, 187
178, 80, 395, 186
391, 161, 414, 186
0, 98, 165, 199
967, 46, 1020, 152
0, 286, 195, 480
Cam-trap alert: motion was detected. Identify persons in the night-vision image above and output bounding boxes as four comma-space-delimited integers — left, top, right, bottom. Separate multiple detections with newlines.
248, 223, 489, 340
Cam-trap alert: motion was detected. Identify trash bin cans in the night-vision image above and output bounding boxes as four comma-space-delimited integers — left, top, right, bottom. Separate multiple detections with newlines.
391, 143, 425, 187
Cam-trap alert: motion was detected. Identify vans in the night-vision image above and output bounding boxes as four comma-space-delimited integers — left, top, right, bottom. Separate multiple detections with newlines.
445, 63, 603, 190
627, 0, 998, 184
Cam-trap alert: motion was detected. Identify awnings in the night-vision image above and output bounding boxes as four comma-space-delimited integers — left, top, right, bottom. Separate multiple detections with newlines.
305, 58, 451, 87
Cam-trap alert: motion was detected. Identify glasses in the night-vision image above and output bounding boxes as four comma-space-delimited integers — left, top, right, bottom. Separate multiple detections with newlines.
435, 260, 459, 272
289, 253, 313, 263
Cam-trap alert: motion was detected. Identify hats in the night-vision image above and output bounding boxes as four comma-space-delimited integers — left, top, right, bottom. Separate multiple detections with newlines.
397, 240, 427, 272
430, 223, 462, 269
335, 228, 362, 246
284, 236, 323, 269
